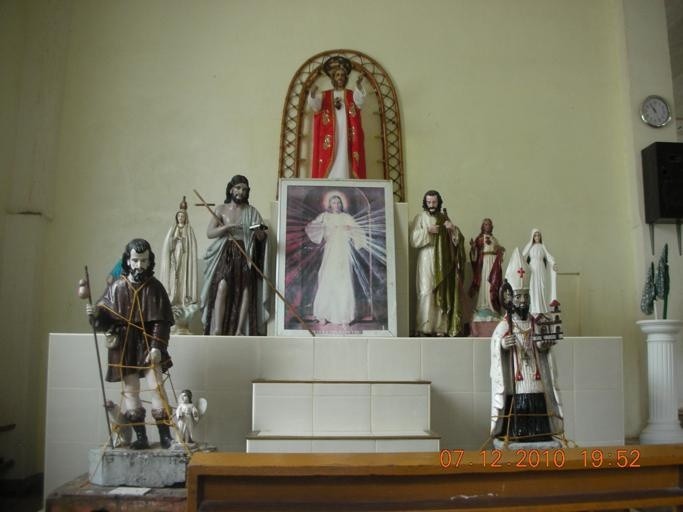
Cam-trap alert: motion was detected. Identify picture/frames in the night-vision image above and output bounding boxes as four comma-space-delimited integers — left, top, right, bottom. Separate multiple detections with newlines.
270, 175, 400, 339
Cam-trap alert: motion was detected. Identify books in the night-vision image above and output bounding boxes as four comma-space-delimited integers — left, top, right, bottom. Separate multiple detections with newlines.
248, 224, 268, 233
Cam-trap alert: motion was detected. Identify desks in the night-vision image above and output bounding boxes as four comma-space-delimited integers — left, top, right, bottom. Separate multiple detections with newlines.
182, 437, 682, 511
42, 472, 191, 510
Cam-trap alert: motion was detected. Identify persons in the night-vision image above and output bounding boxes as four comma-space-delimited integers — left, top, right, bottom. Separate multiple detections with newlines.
306, 63, 367, 180
525, 228, 559, 316
302, 195, 367, 331
198, 173, 271, 335
469, 216, 506, 317
410, 188, 467, 337
488, 287, 564, 442
85, 236, 178, 450
157, 211, 200, 310
174, 386, 199, 445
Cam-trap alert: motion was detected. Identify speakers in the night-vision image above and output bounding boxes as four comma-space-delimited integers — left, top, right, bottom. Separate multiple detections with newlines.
641, 141, 683, 224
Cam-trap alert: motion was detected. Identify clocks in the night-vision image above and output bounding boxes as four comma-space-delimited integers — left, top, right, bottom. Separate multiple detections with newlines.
640, 91, 673, 131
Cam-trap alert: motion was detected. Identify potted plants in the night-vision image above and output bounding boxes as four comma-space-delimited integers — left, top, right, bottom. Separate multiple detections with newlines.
634, 243, 683, 444
